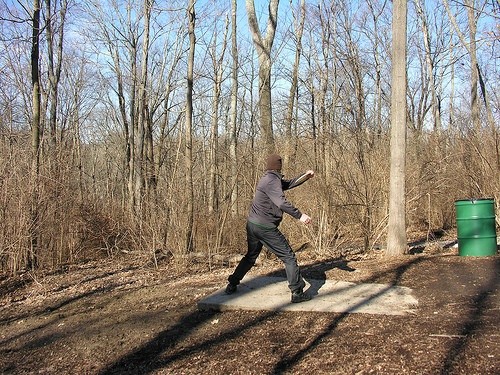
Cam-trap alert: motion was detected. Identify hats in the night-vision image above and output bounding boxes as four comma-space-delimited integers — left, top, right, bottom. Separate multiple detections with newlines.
267, 153, 282, 170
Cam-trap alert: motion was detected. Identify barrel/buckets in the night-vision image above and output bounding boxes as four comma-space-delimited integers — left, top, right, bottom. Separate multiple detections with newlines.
454, 197, 498, 257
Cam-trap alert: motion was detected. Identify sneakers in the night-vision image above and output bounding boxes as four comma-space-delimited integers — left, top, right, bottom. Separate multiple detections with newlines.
291, 292, 311, 303
226, 284, 237, 294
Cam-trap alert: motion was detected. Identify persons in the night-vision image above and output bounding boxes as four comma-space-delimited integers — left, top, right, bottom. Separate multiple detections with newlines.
225, 153, 315, 303
